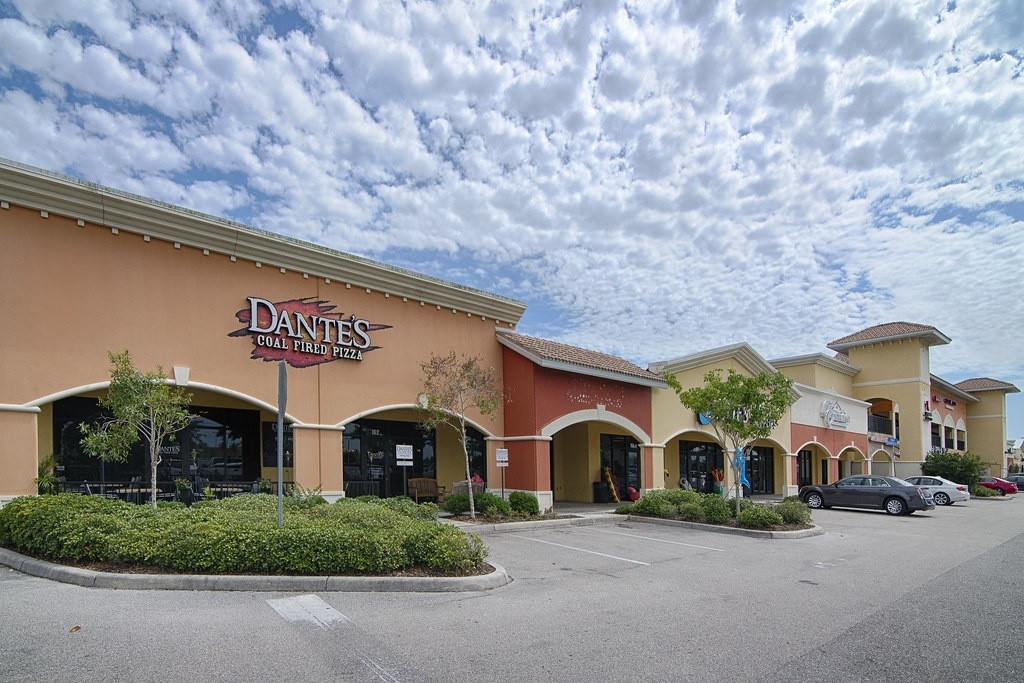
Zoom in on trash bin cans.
[592,481,610,504]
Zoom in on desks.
[202,488,243,499]
[117,489,162,505]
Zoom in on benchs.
[442,480,484,501]
[408,478,446,504]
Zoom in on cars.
[1001,475,1024,491]
[901,476,970,505]
[977,476,1018,497]
[798,474,936,516]
